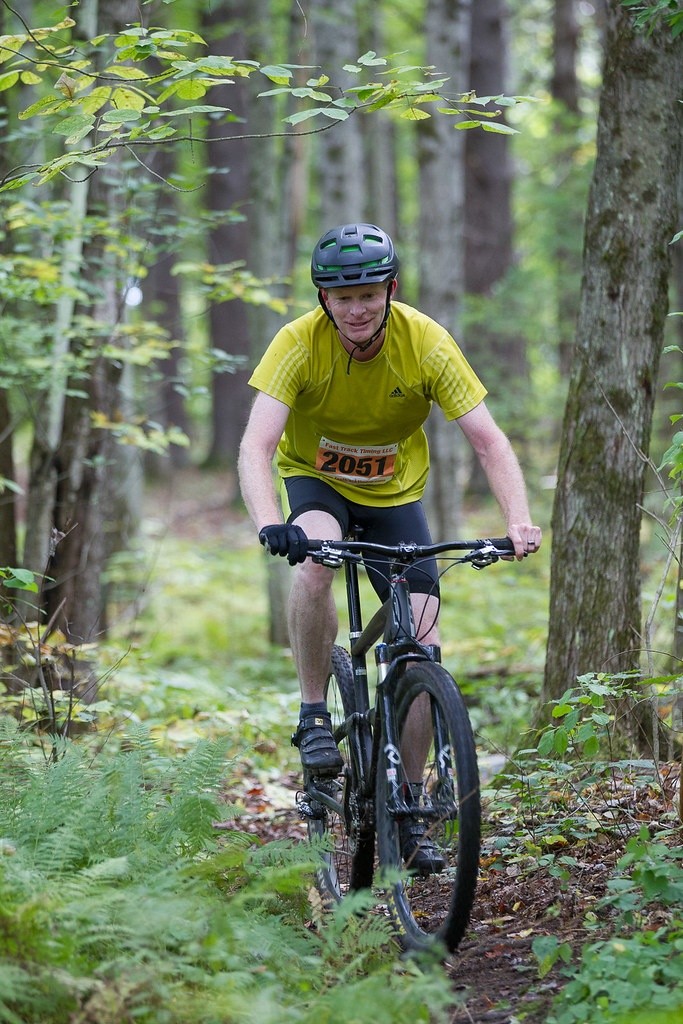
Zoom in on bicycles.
[260,524,539,961]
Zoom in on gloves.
[259,524,308,566]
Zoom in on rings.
[529,541,535,545]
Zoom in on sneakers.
[290,709,344,769]
[398,813,444,873]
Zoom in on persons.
[238,224,541,874]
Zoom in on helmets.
[311,223,401,289]
